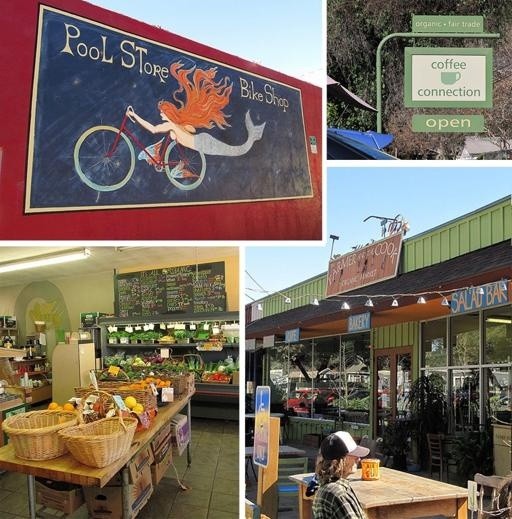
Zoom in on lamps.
[262,280,511,311]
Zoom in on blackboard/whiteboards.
[114,261,226,317]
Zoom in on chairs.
[427,433,457,482]
[273,457,308,519]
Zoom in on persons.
[311,430,368,519]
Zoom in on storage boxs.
[34,414,189,519]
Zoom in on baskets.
[73,367,196,419]
[57,389,138,467]
[1,409,78,459]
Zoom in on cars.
[278,370,413,422]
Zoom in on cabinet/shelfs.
[0,327,52,446]
[96,312,239,420]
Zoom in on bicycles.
[70,102,210,195]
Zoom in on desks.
[244,412,285,421]
[0,388,196,519]
[288,467,480,519]
[245,446,306,481]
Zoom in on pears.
[123,396,143,415]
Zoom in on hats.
[320,430,370,459]
